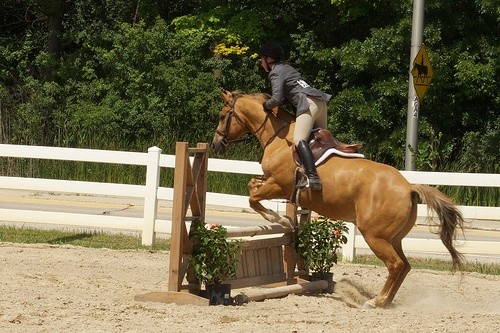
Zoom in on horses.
[211,88,472,308]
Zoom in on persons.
[258,42,332,190]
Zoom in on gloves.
[262,100,274,113]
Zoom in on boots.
[295,138,322,190]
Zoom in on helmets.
[258,42,285,60]
